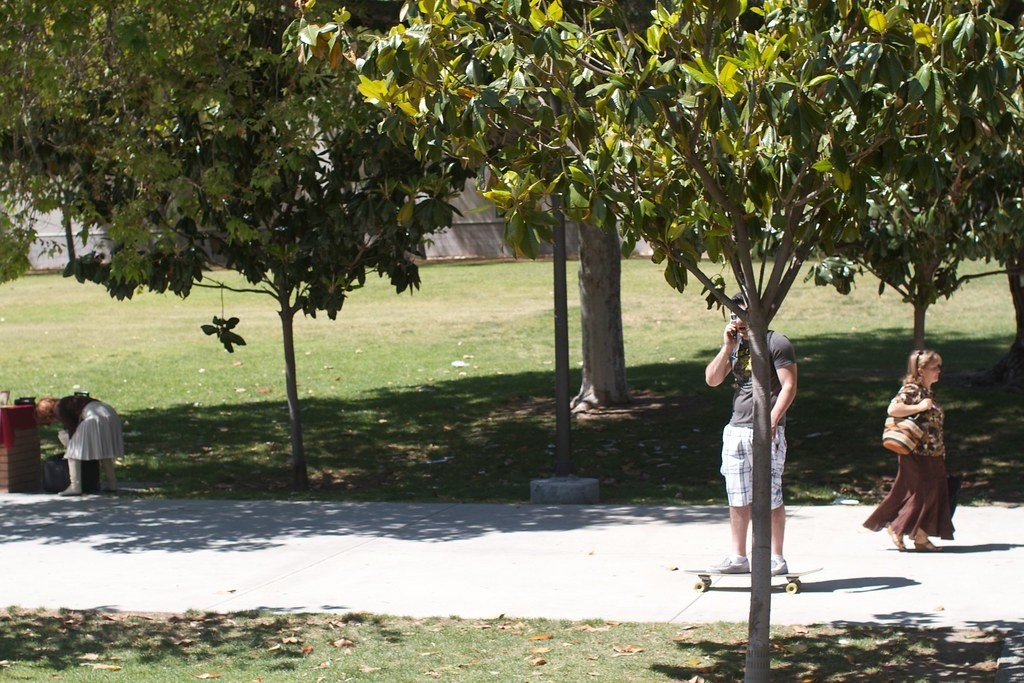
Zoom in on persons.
[34,395,123,496]
[705,291,798,574]
[886,350,950,553]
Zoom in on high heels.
[914,540,943,552]
[887,524,907,551]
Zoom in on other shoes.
[706,556,750,574]
[101,484,118,494]
[770,558,788,576]
[58,486,82,496]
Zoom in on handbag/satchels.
[882,386,924,455]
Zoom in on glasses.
[915,349,924,376]
[737,326,747,333]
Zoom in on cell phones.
[732,326,737,337]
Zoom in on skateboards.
[672,565,824,594]
